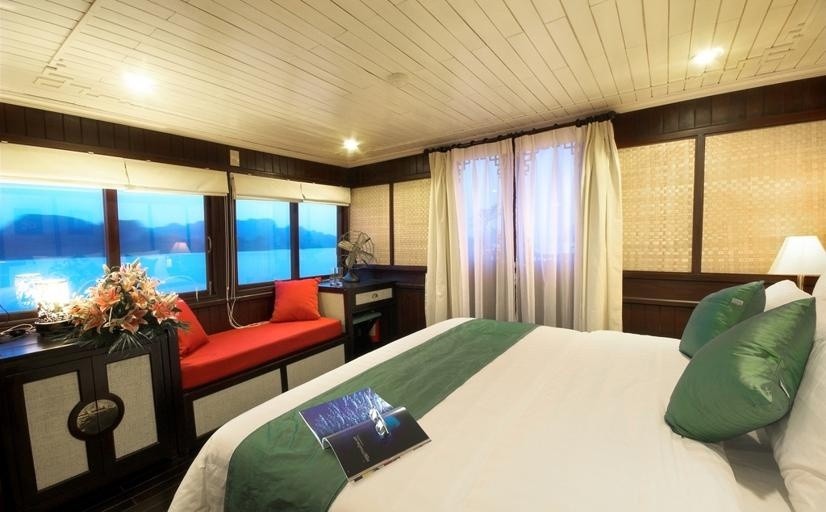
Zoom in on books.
[299,386,431,484]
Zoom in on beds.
[167,273,826,512]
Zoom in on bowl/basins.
[32,318,73,336]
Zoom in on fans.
[335,231,375,283]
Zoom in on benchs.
[178,316,352,468]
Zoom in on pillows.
[679,279,766,358]
[173,298,210,360]
[664,297,816,444]
[268,277,322,324]
[764,339,826,512]
[764,279,812,311]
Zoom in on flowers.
[63,259,192,356]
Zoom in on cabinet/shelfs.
[0,312,180,510]
[318,276,399,363]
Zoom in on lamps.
[767,236,826,291]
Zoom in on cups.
[333,267,345,285]
[328,276,336,286]
